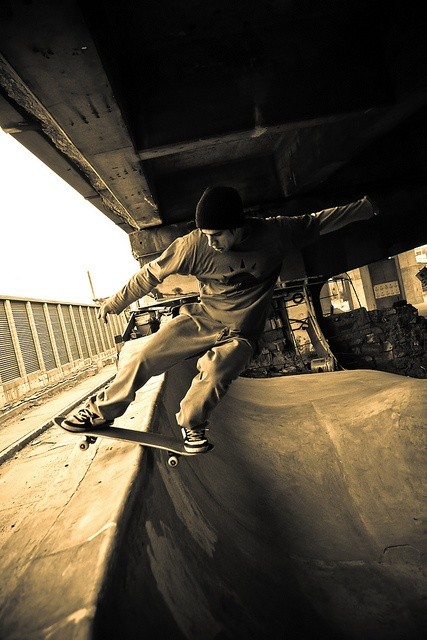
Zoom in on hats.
[194,183,247,231]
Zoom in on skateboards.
[52,417,216,468]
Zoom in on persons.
[60,182,380,453]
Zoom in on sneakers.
[61,403,116,433]
[181,422,210,453]
